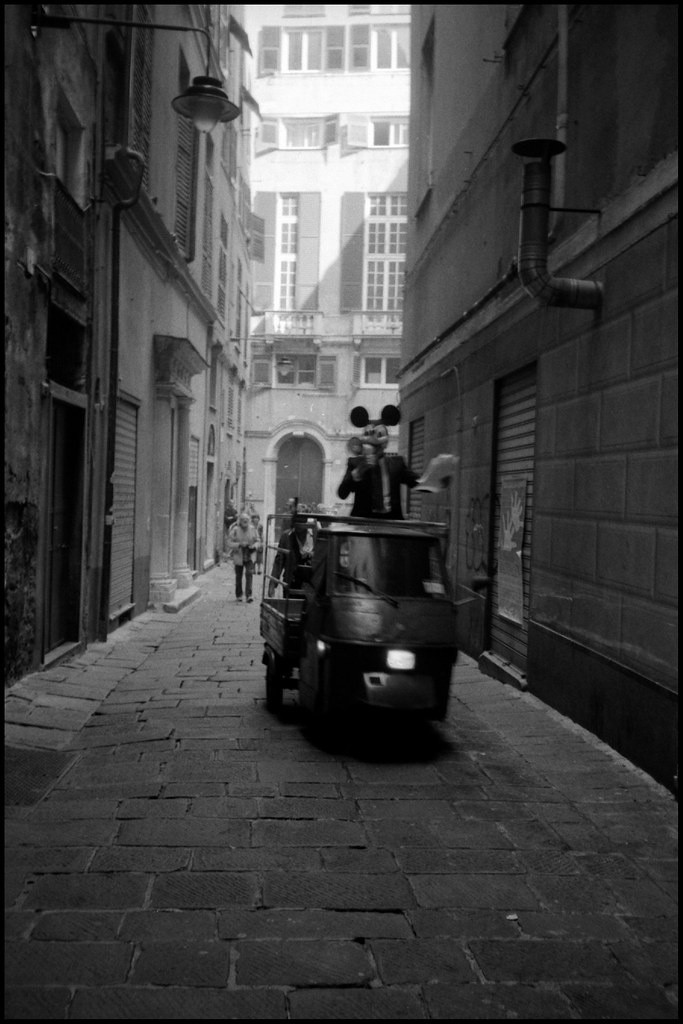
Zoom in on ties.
[379,458,391,512]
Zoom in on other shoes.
[237,597,242,601]
[246,596,253,603]
[257,571,261,575]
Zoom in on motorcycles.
[259,511,459,766]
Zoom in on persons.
[337,404,452,521]
[251,512,263,575]
[226,513,261,603]
[224,499,237,529]
[281,498,329,531]
[267,516,314,598]
